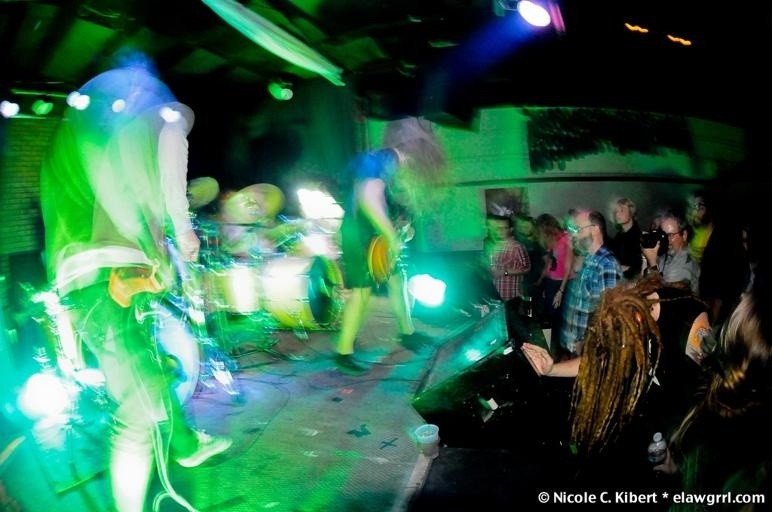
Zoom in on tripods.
[193,219,310,405]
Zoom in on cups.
[415,423,441,460]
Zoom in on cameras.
[641,229,669,257]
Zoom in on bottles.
[647,431,668,480]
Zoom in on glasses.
[668,229,682,240]
[578,224,595,233]
[695,202,706,210]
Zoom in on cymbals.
[226,183,284,231]
[186,175,219,209]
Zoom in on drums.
[211,260,260,318]
[258,251,343,337]
[228,232,276,264]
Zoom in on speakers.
[410,296,552,449]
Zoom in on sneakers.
[176,432,231,468]
[336,354,367,377]
[401,333,434,345]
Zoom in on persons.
[404,173,772,512]
[36,8,232,511]
[331,117,443,374]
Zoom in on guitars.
[367,212,413,287]
